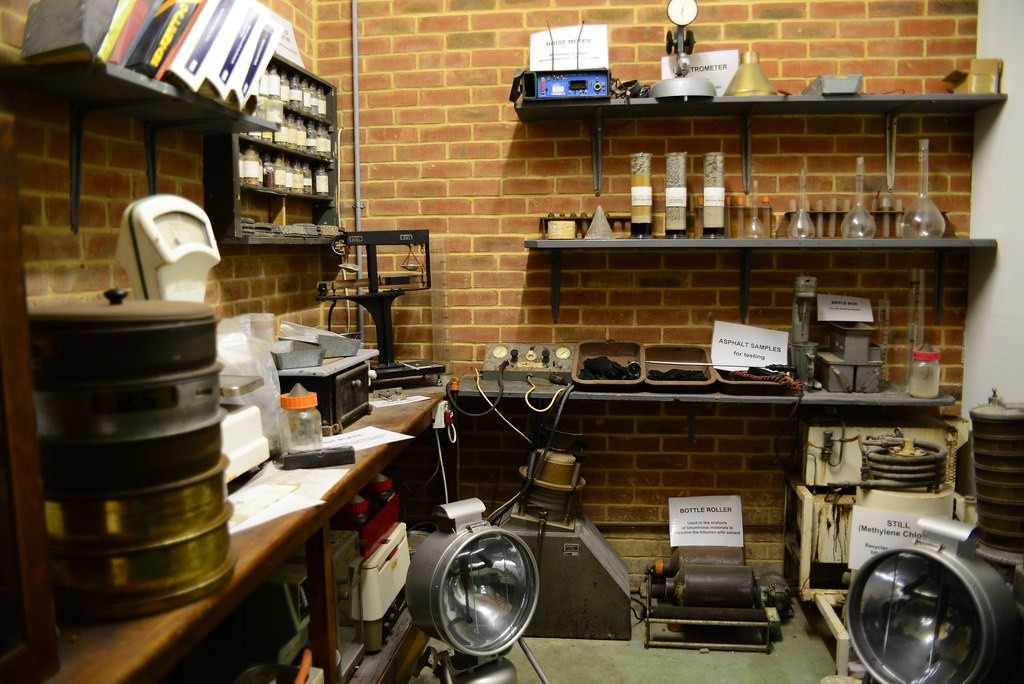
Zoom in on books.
[20,1,283,115]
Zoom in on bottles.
[840,157,876,238]
[240,63,332,197]
[786,168,815,238]
[744,179,766,237]
[909,343,941,398]
[277,383,323,456]
[901,139,946,239]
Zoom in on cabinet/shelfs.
[202,52,340,245]
[523,89,1008,322]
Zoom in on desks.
[53,374,453,684]
[456,371,956,584]
